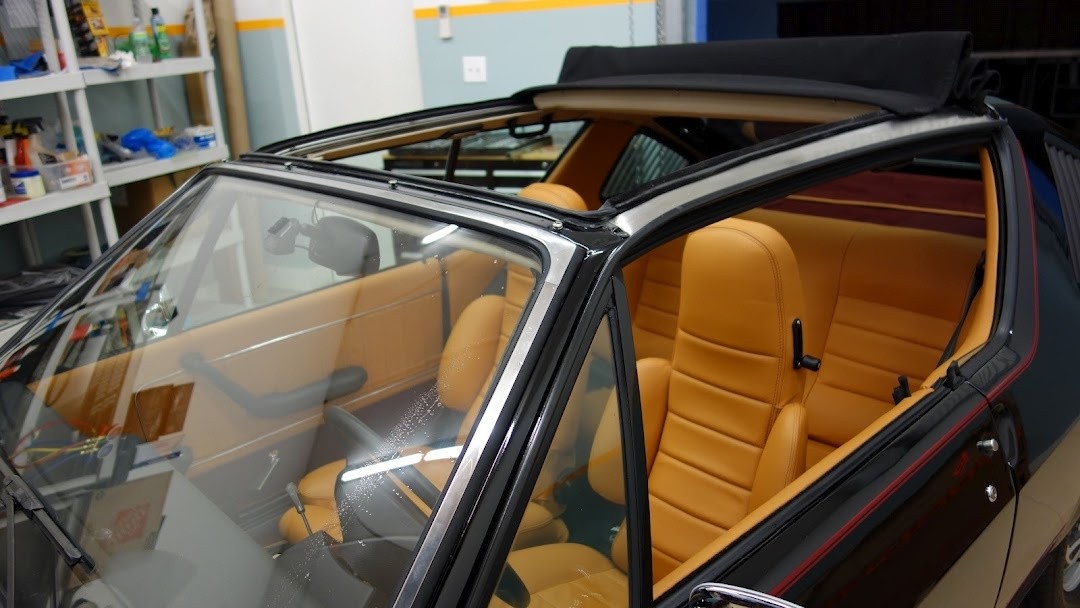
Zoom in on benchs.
[577,179,993,467]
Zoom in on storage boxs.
[128,164,199,221]
[67,0,109,59]
[36,150,95,191]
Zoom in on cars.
[0,30,1079,608]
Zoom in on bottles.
[9,170,48,199]
[129,17,153,65]
[150,8,172,60]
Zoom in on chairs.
[486,217,820,608]
[278,181,617,567]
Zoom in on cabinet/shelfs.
[0,0,252,357]
